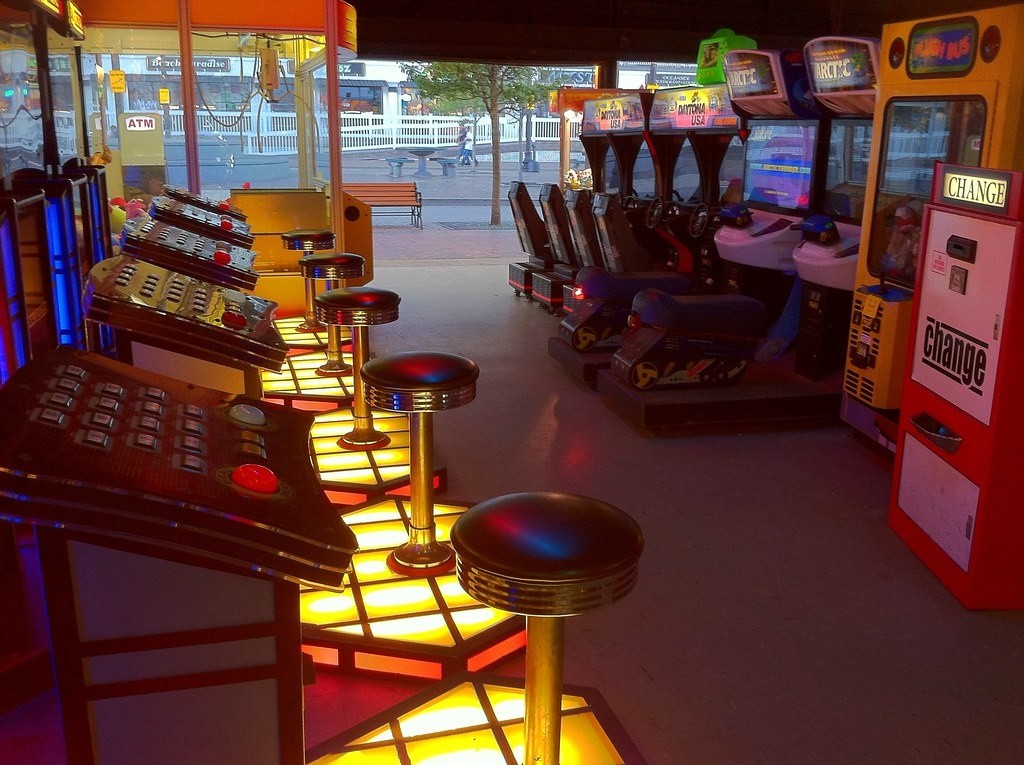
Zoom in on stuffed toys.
[875,206,920,281]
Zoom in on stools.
[298,253,366,378]
[448,491,648,764]
[311,286,403,452]
[385,158,416,178]
[360,350,481,579]
[429,158,458,177]
[281,228,338,335]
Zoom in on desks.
[405,147,439,178]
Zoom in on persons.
[457,126,478,166]
[455,123,467,162]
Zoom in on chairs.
[562,186,603,271]
[538,183,580,268]
[591,191,670,286]
[507,181,550,258]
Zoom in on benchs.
[341,181,424,229]
